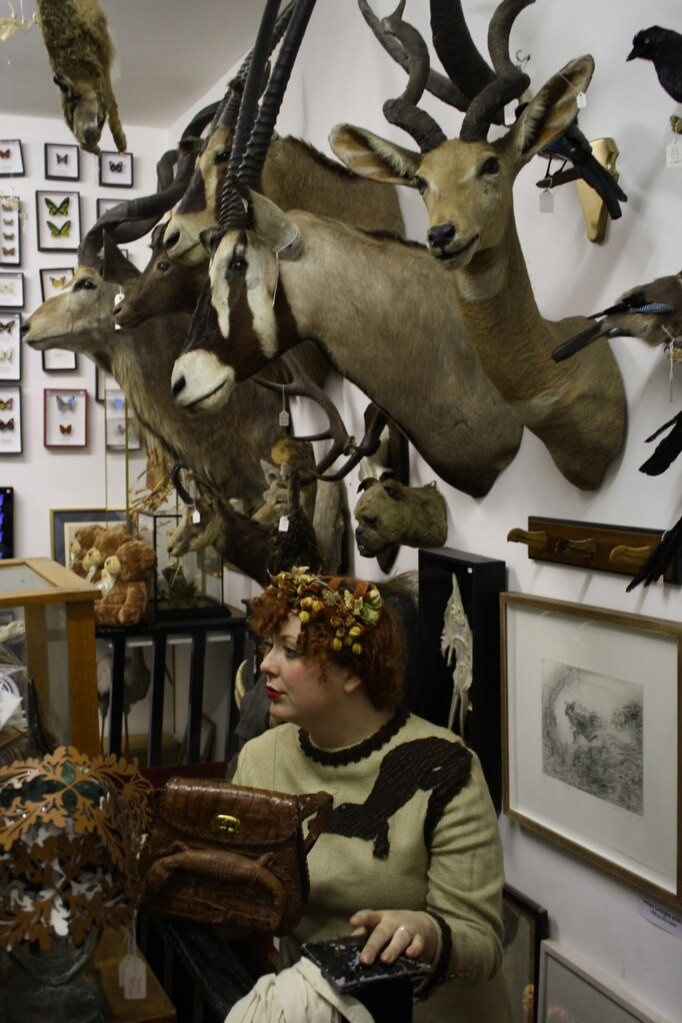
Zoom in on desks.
[94,598,245,767]
[0,555,103,770]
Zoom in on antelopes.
[20,0,682,589]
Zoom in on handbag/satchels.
[131,777,333,936]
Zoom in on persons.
[231,576,514,1022]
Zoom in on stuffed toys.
[71,523,155,627]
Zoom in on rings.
[402,926,412,936]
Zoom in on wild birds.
[624,24,682,105]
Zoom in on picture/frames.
[96,198,128,220]
[501,881,676,1023]
[0,385,23,454]
[44,142,80,181]
[0,196,21,265]
[0,139,24,175]
[98,150,133,186]
[104,388,141,451]
[0,248,130,401]
[497,591,682,908]
[35,190,81,250]
[43,388,87,448]
[49,508,139,567]
